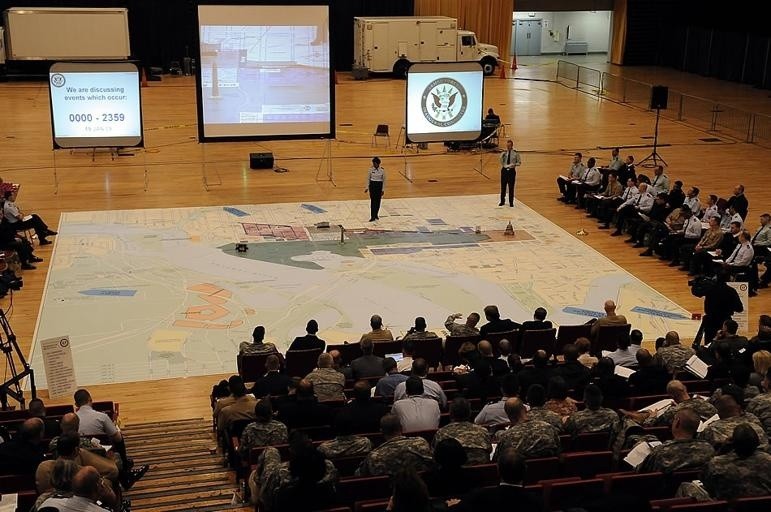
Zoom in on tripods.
[634,109,668,167]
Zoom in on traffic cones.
[138,68,149,86]
[508,54,519,70]
[499,64,506,79]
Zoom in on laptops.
[384,352,404,366]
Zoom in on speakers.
[250,153,274,170]
[650,84,668,109]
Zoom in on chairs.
[212,323,771,512]
[732,255,759,295]
[372,125,390,148]
[0,210,34,272]
[0,401,120,512]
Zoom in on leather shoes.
[40,231,57,246]
[21,256,42,269]
[556,198,701,276]
[122,464,149,490]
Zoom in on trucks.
[352,14,501,80]
[0,4,131,81]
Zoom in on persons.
[288,320,325,352]
[703,271,744,348]
[365,157,386,222]
[402,317,438,341]
[1,190,58,301]
[480,306,521,339]
[349,340,447,435]
[452,339,594,428]
[353,395,562,511]
[1,388,149,512]
[253,411,373,510]
[348,338,388,378]
[573,314,770,418]
[212,352,349,468]
[499,140,522,207]
[444,312,481,337]
[607,396,770,500]
[562,382,622,435]
[585,300,627,336]
[360,314,393,344]
[558,147,770,295]
[485,108,501,136]
[238,326,279,355]
[523,307,553,330]
